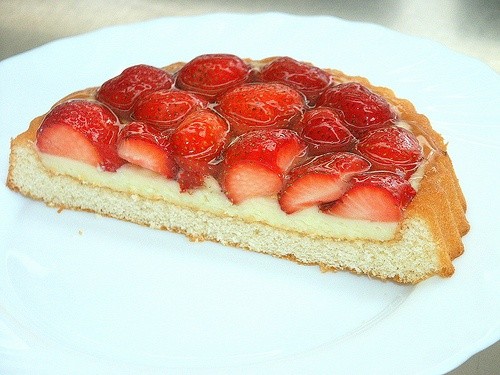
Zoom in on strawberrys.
[36,53,422,222]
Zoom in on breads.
[4,57,470,286]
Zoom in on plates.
[0,10,499,375]
